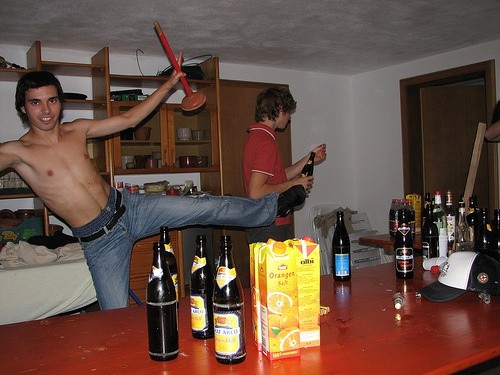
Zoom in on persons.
[243,86,326,245]
[0,49,305,310]
[484,120,500,142]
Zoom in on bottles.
[389,199,416,279]
[159,226,180,309]
[212,233,246,364]
[421,191,500,262]
[190,235,214,340]
[422,256,448,270]
[393,292,406,309]
[431,262,449,276]
[146,241,180,361]
[300,152,315,178]
[332,211,351,282]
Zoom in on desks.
[0,234,500,375]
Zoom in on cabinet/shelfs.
[0,39,226,306]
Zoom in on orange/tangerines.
[267,292,300,352]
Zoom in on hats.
[419,250,500,303]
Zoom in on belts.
[74,188,127,243]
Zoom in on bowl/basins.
[144,183,166,193]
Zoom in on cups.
[133,127,151,141]
[122,153,208,169]
[177,127,206,141]
[166,186,197,196]
[114,182,146,194]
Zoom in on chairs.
[310,204,388,274]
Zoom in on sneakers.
[276,183,306,217]
